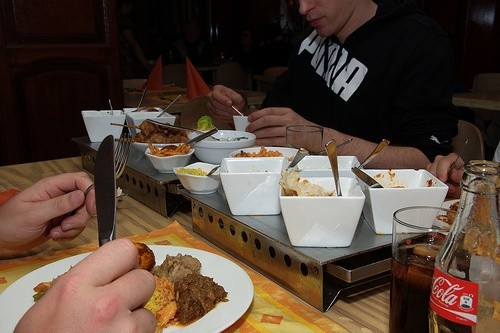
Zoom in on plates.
[0,244,254,332]
[432,199,460,229]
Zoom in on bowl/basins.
[293,155,365,170]
[279,170,366,247]
[81,111,126,143]
[123,106,176,139]
[228,147,299,163]
[173,161,221,195]
[232,116,250,131]
[187,130,256,164]
[134,142,182,155]
[356,168,450,234]
[144,146,195,173]
[220,158,288,215]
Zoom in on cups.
[388,206,457,333]
[286,125,324,156]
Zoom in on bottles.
[428,159,500,333]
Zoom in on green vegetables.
[196,115,216,130]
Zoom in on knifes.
[94,134,116,248]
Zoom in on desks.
[454,88,500,127]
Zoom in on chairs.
[458,118,483,163]
[474,72,500,120]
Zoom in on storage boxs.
[77,104,452,250]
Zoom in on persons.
[0,172,159,333]
[118,0,500,199]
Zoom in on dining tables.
[0,152,405,333]
